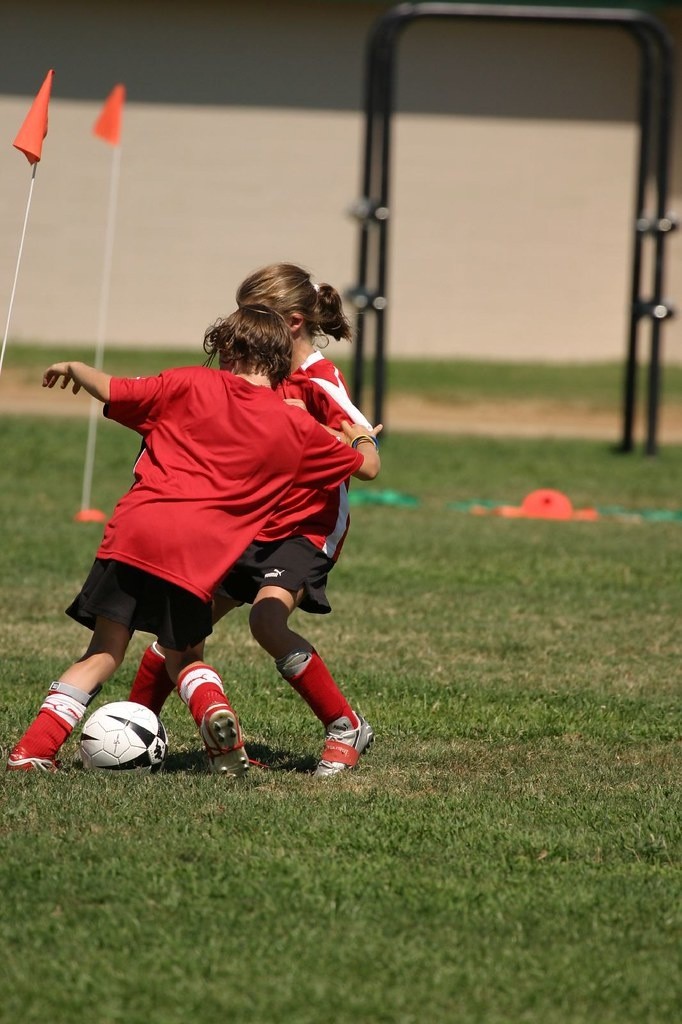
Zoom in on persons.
[7,304,382,771]
[129,264,374,774]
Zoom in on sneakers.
[6,744,69,776]
[200,700,251,779]
[314,710,374,776]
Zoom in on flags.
[93,83,126,144]
[13,70,53,165]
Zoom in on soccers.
[77,700,170,774]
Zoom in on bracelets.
[350,434,380,453]
